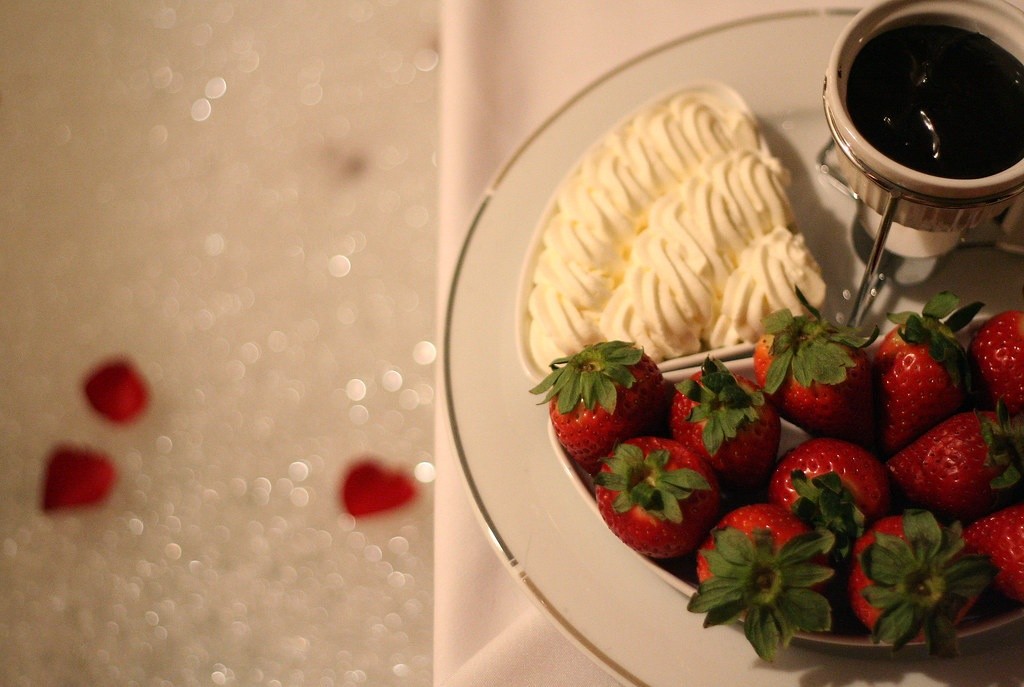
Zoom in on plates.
[441,11,1021,686]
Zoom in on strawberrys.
[528,293,1023,661]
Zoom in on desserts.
[519,82,826,384]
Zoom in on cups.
[822,1,1023,260]
